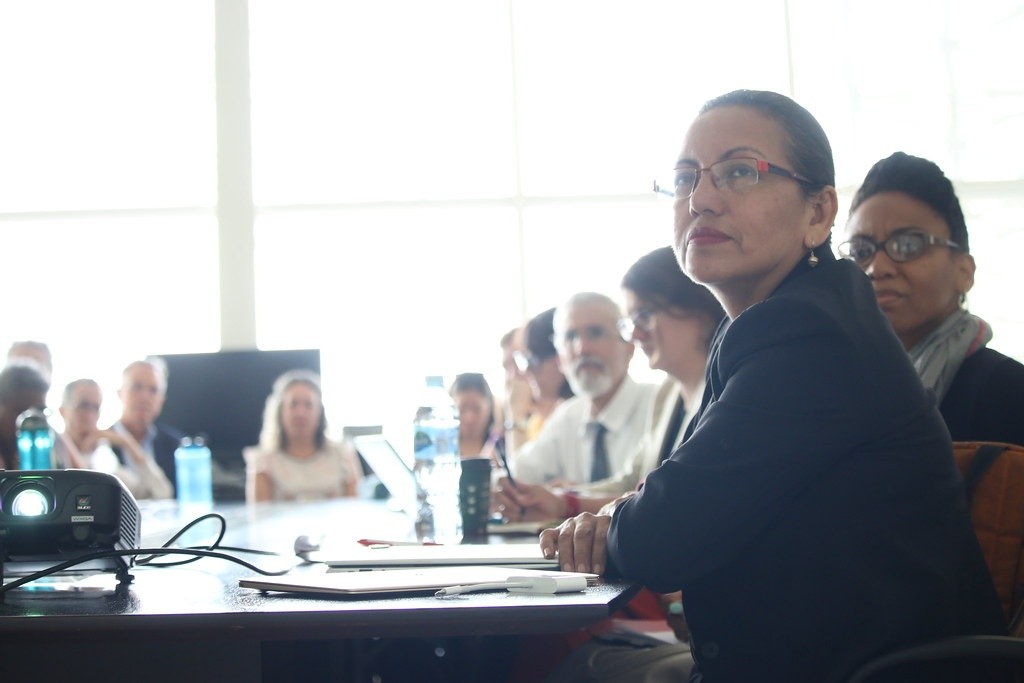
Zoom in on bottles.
[413,375,464,546]
[174,436,214,509]
[13,403,51,470]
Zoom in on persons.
[0,342,365,506]
[451,91,1024,683]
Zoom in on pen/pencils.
[358,538,443,546]
[495,439,525,517]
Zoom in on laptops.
[353,434,544,533]
[326,543,563,573]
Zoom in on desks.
[0,500,644,683]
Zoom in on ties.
[590,422,610,483]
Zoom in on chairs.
[849,634,1024,683]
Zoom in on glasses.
[619,299,686,342]
[63,401,99,414]
[652,155,817,200]
[836,229,961,269]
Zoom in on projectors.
[0,467,142,582]
